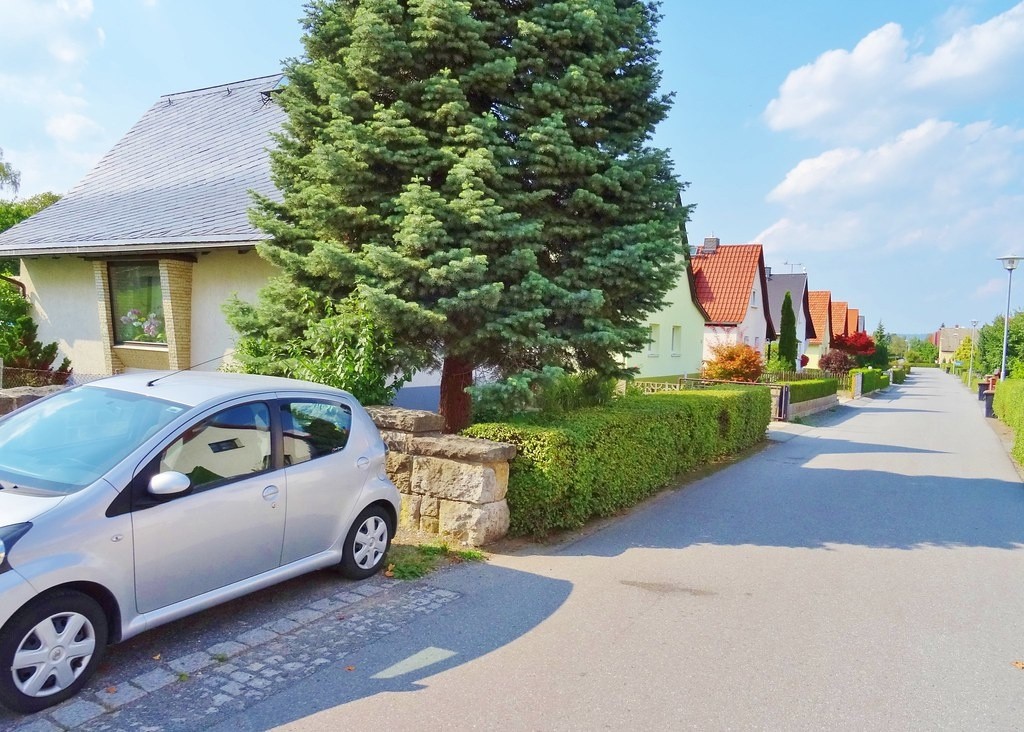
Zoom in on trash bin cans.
[978,382,990,401]
[983,389,996,418]
[946,367,951,373]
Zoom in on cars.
[0,368,402,710]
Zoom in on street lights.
[996,254,1024,382]
[968,319,980,392]
[953,333,959,374]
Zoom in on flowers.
[120,309,162,337]
